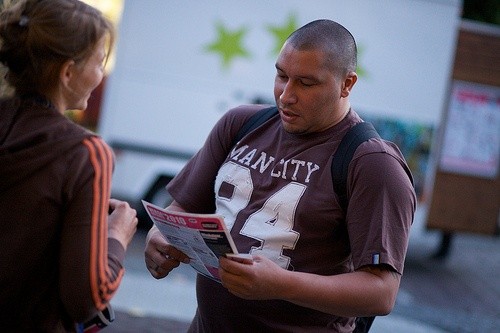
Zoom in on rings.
[149,264,160,273]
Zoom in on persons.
[139,18,416,332]
[0,0,136,333]
